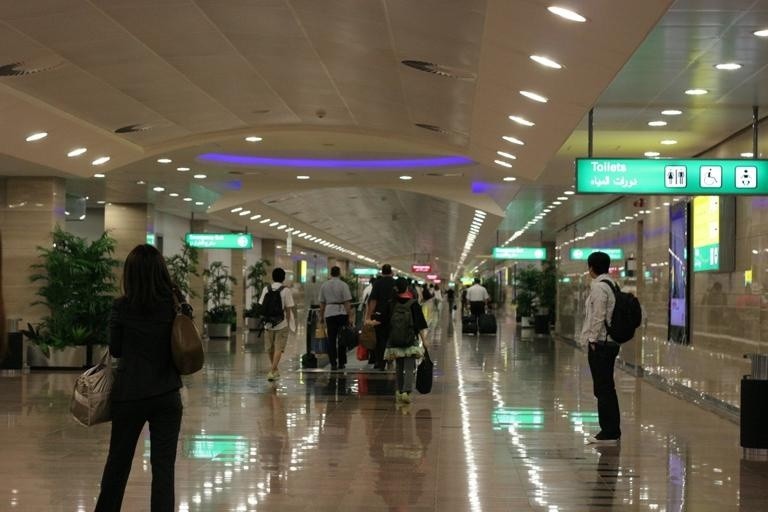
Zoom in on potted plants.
[203,259,236,339]
[243,256,274,329]
[17,223,122,371]
[516,265,568,335]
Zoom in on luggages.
[463,309,477,333]
[479,305,496,334]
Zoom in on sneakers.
[396,390,411,403]
[268,370,279,381]
[588,431,620,444]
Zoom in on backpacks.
[600,279,641,343]
[261,285,285,316]
[390,298,417,345]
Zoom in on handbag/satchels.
[372,312,390,332]
[416,360,432,394]
[171,313,204,374]
[71,361,118,428]
[315,328,328,339]
[361,327,376,350]
[357,346,369,360]
[344,327,358,350]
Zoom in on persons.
[578,250,624,447]
[445,286,454,312]
[255,266,295,382]
[358,264,434,404]
[94,243,183,512]
[460,277,492,317]
[434,284,442,311]
[318,266,354,370]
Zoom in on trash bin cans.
[0,317,24,378]
[534,306,550,338]
[740,352,768,462]
